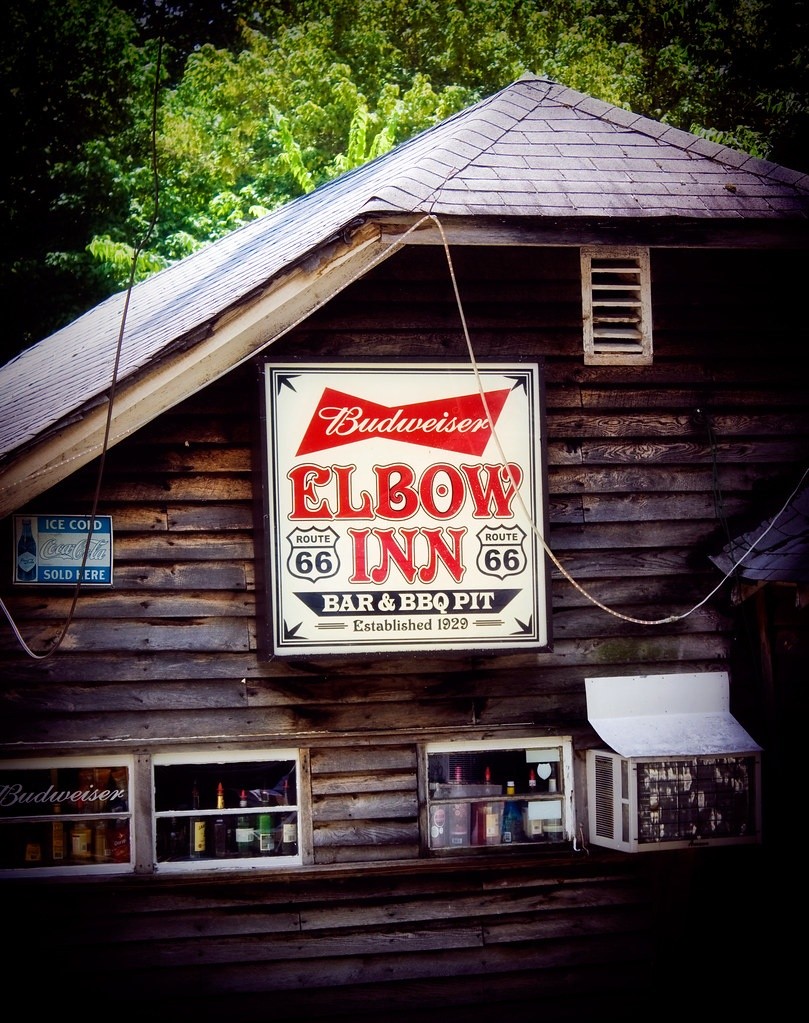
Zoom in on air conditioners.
[586,747,762,853]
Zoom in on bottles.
[474,768,502,845]
[523,769,543,842]
[502,781,523,844]
[277,781,297,855]
[213,783,229,857]
[258,792,274,854]
[545,763,563,840]
[24,801,129,861]
[234,790,254,853]
[449,767,470,847]
[75,768,128,811]
[430,782,447,846]
[189,789,206,860]
